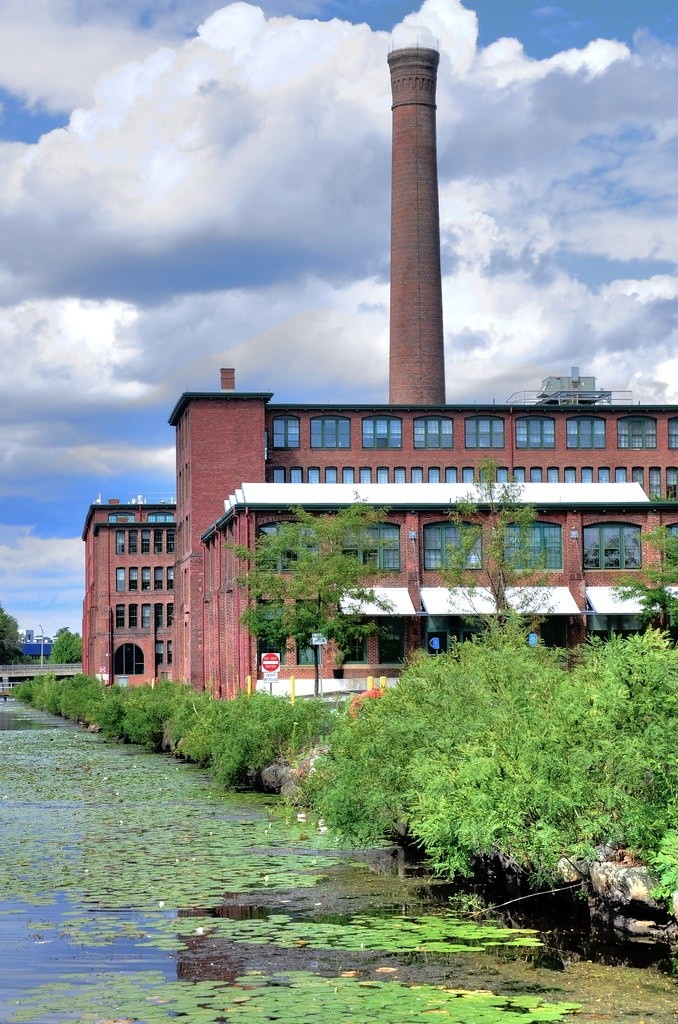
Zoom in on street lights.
[38,624,44,671]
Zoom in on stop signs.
[261,652,281,673]
